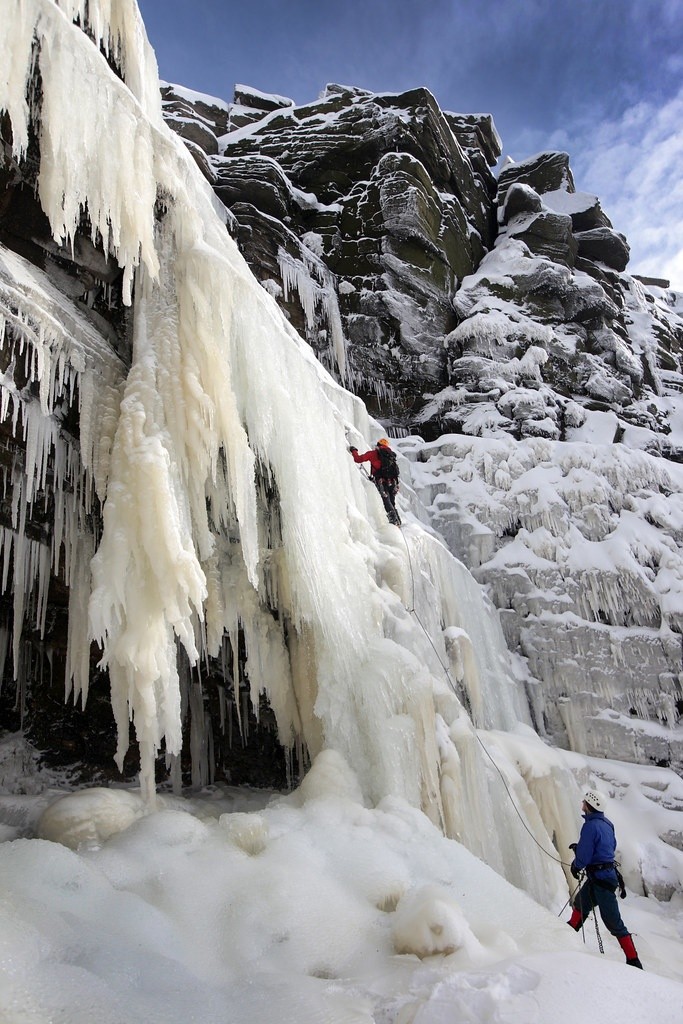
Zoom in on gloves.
[349,446,358,452]
[570,859,580,880]
[568,843,579,857]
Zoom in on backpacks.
[376,449,400,485]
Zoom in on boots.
[386,509,401,526]
[566,904,588,933]
[616,932,643,970]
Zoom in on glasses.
[376,442,382,446]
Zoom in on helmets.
[378,438,389,446]
[583,790,607,813]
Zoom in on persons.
[567,790,647,970]
[345,438,409,528]
